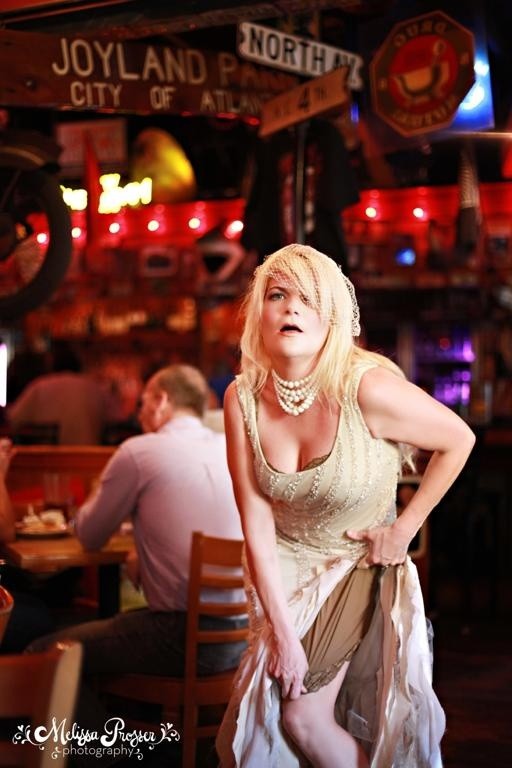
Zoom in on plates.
[17,523,71,539]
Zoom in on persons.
[76,364,246,757]
[211,243,476,768]
[8,344,105,449]
[0,430,18,543]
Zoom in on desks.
[3,533,138,620]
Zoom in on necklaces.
[270,367,323,415]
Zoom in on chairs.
[0,640,87,767]
[100,529,256,767]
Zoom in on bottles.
[412,331,478,426]
